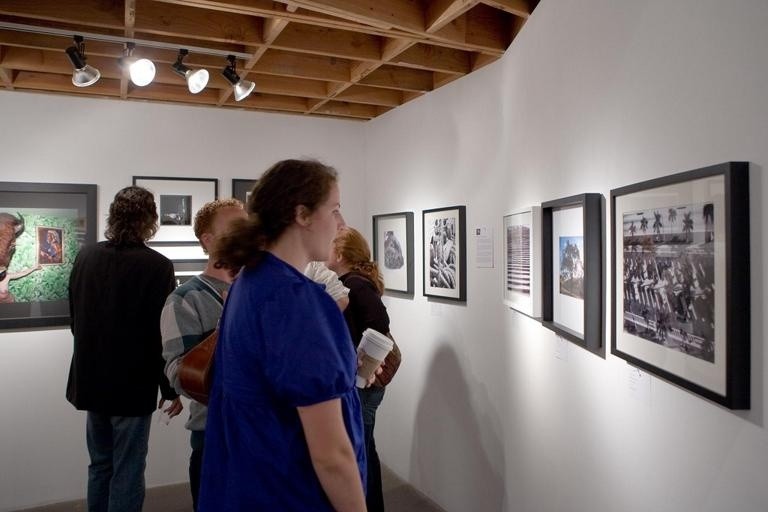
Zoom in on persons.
[41,228,63,264]
[197,157,365,511]
[304,257,350,313]
[64,182,184,511]
[322,224,401,510]
[160,197,247,511]
[384,230,401,259]
[429,219,456,288]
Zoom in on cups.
[352,328,394,389]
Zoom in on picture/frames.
[500,206,543,321]
[231,178,262,207]
[370,211,414,299]
[539,191,603,353]
[33,224,65,268]
[422,206,468,305]
[610,160,752,410]
[132,176,220,247]
[172,259,217,288]
[0,180,101,328]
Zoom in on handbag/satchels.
[177,326,222,407]
[371,329,402,390]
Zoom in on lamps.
[65,31,256,103]
